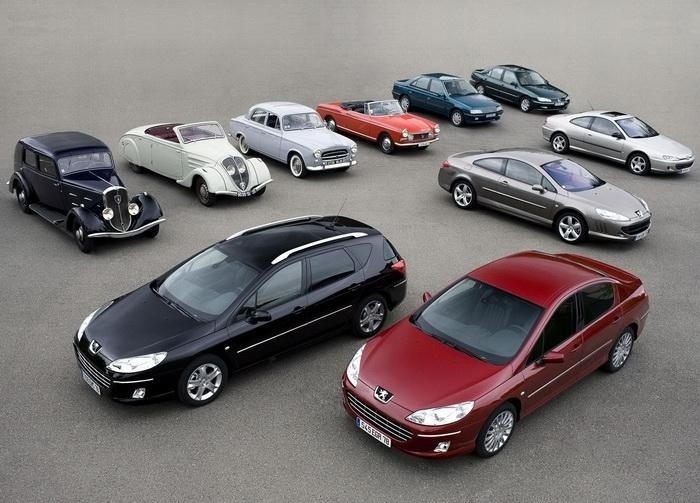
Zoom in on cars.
[541,109,695,175]
[437,146,652,243]
[391,73,502,126]
[341,251,651,461]
[74,214,406,408]
[8,128,167,253]
[228,101,359,178]
[469,64,568,111]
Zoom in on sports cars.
[118,113,274,206]
[316,98,441,154]
